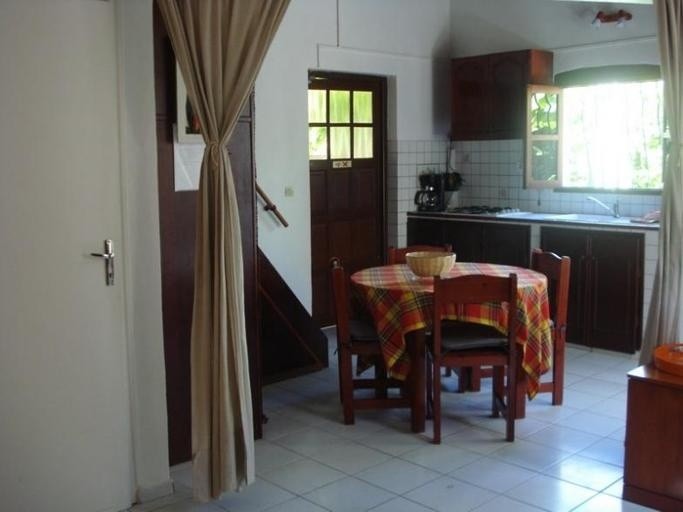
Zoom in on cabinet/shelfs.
[451,49,554,141]
[153,0,262,466]
[407,219,530,268]
[541,227,645,354]
[622,363,683,512]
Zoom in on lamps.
[590,10,632,29]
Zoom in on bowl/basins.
[405,250,457,280]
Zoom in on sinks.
[545,213,632,224]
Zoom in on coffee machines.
[415,173,444,211]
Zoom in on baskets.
[406,251,456,276]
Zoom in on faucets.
[587,196,621,218]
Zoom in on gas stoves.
[448,205,521,214]
